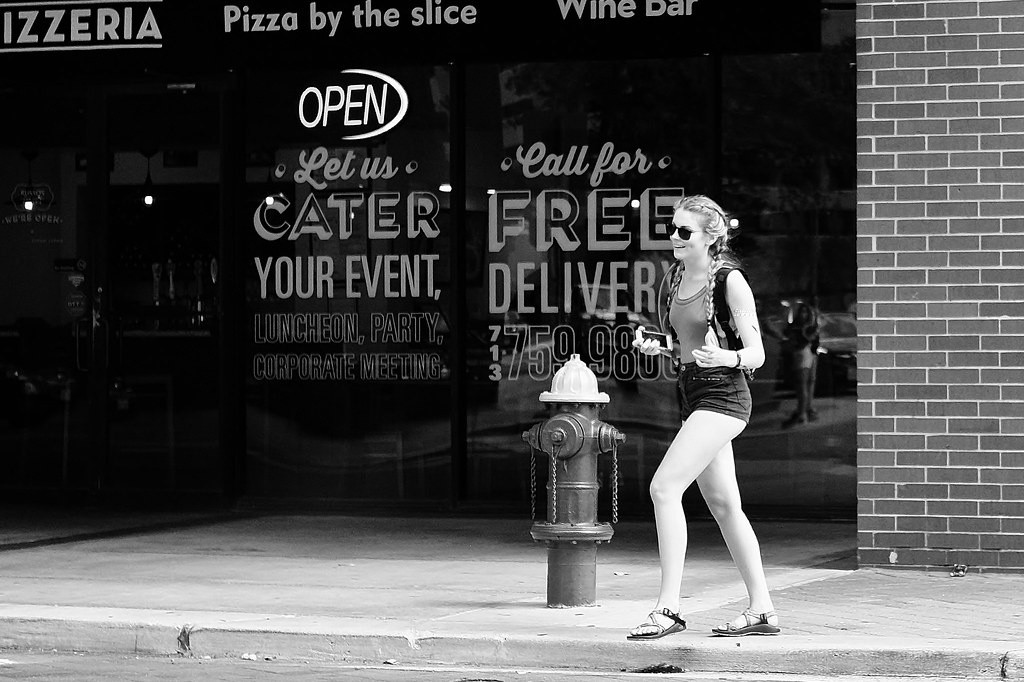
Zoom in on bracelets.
[731,350,741,368]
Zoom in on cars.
[810,313,857,396]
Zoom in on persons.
[626,194,780,641]
[781,283,819,429]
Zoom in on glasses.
[666,223,705,240]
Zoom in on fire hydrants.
[522,354,627,610]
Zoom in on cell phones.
[635,330,674,352]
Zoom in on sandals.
[626,608,686,640]
[711,609,780,637]
